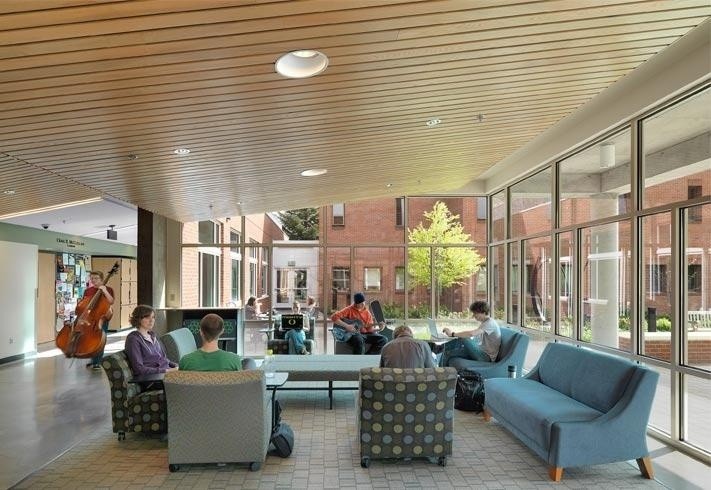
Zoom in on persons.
[245,297,263,320]
[178,313,256,372]
[125,305,178,390]
[306,295,315,313]
[279,301,311,355]
[331,292,388,355]
[428,301,502,367]
[85,271,115,370]
[379,325,437,369]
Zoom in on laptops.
[282,314,303,330]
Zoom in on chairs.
[359,365,457,469]
[333,323,371,355]
[267,317,315,355]
[446,328,530,379]
[100,327,273,473]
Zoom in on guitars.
[332,318,394,344]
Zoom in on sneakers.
[86,363,101,369]
[427,341,443,354]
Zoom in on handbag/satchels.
[270,423,294,457]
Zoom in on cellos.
[56,262,119,359]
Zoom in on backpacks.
[454,369,485,412]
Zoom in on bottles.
[265,349,275,379]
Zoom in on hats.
[354,292,365,304]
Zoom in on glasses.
[92,277,96,279]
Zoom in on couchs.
[482,342,661,482]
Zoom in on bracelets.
[452,331,455,337]
